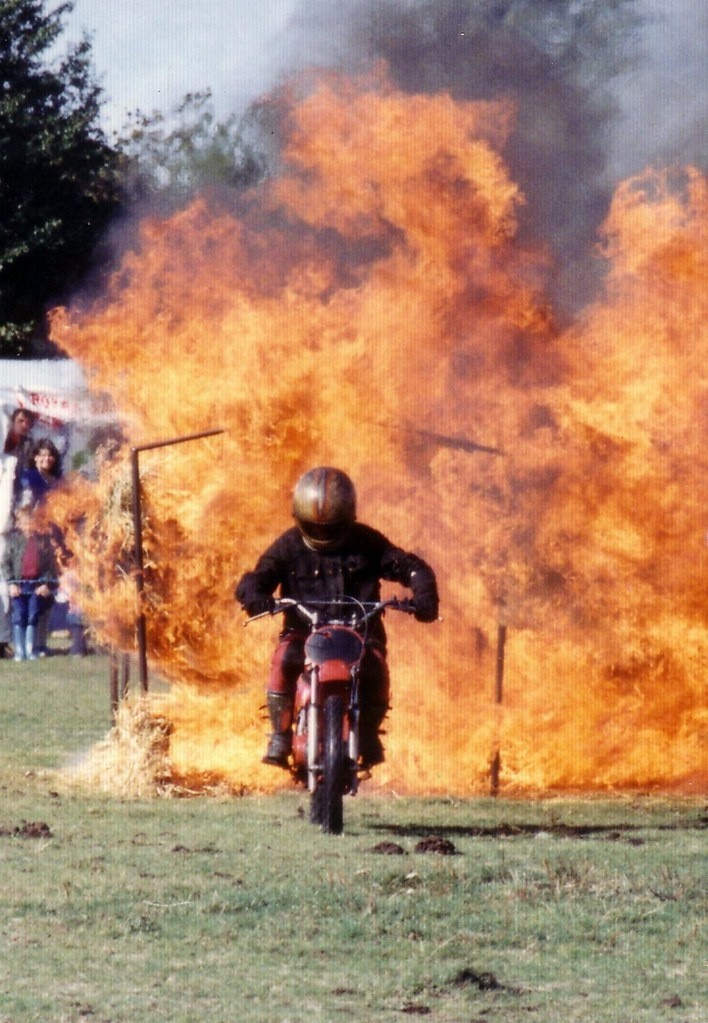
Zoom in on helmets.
[291,466,356,552]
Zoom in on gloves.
[243,587,275,617]
[408,589,439,623]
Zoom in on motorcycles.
[244,590,444,838]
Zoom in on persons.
[0,506,60,661]
[0,409,103,659]
[235,467,439,767]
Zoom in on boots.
[260,689,295,760]
[25,624,37,660]
[12,624,25,661]
[360,699,392,763]
